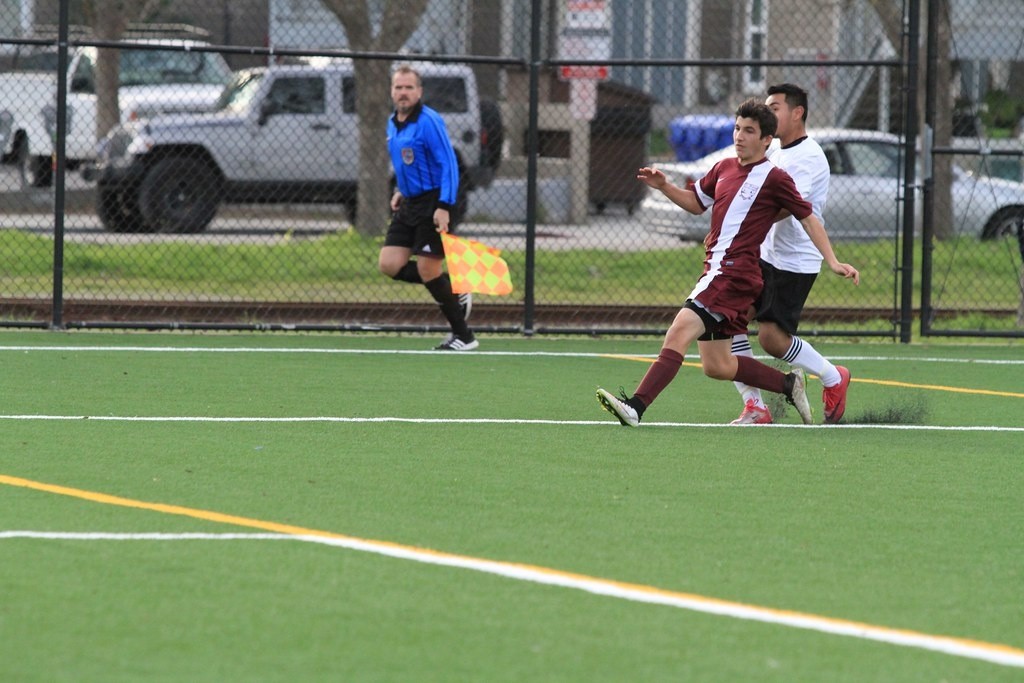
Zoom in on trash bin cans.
[669,114,737,164]
[589,79,658,215]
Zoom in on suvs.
[78,50,504,234]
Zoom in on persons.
[730,83,852,426]
[598,97,859,428]
[382,65,481,352]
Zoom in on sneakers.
[432,330,479,351]
[823,366,850,423]
[458,292,471,322]
[730,398,772,424]
[784,367,814,425]
[596,385,640,428]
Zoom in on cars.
[0,31,234,186]
[637,125,1024,252]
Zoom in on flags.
[442,227,513,298]
